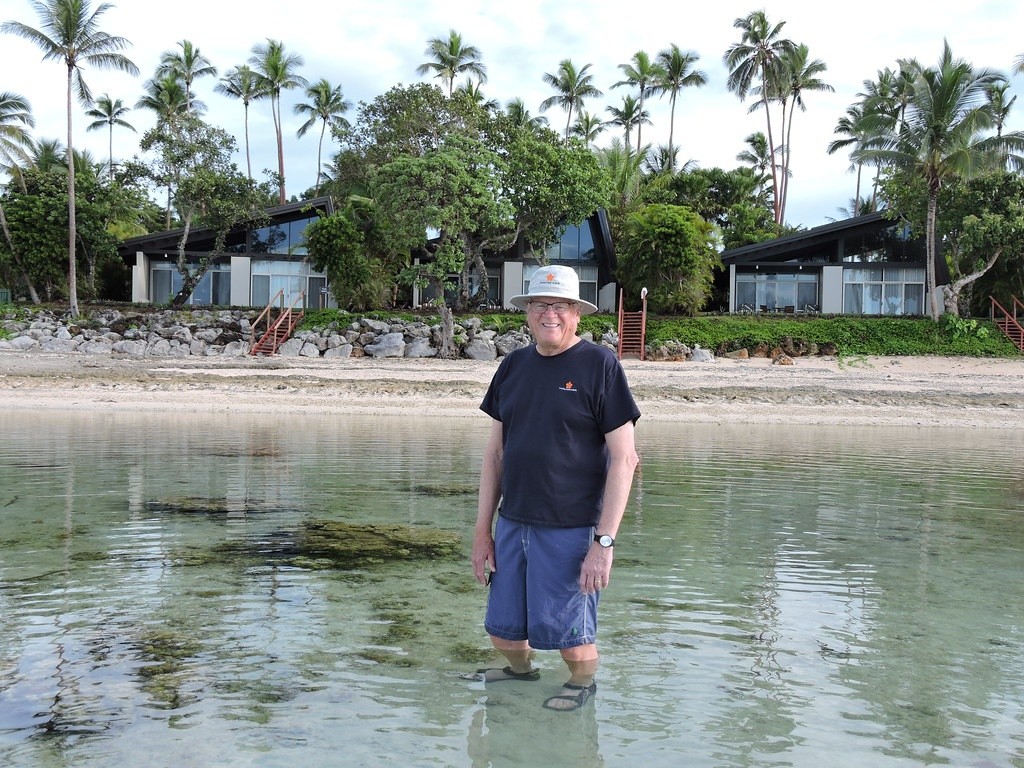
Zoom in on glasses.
[527,298,577,313]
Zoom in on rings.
[594,580,601,583]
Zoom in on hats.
[510,265,598,314]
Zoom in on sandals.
[459,665,540,684]
[542,678,597,713]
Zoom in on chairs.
[782,305,794,314]
[760,305,772,312]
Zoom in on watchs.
[593,531,615,549]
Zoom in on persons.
[460,264,641,713]
